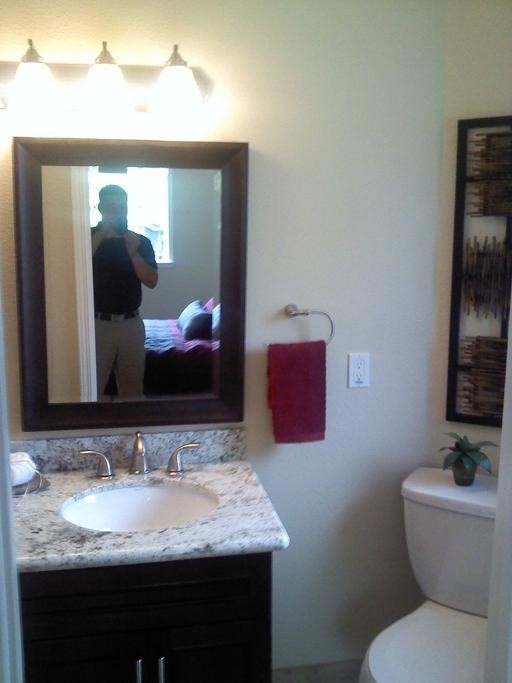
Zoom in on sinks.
[60,479,218,533]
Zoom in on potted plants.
[437,430,501,486]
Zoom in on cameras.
[114,216,127,235]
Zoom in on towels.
[211,303,222,341]
[267,341,326,444]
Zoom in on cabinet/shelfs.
[18,553,272,683]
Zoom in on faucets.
[129,431,150,474]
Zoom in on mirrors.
[11,133,249,433]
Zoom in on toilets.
[357,466,512,683]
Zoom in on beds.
[104,318,221,396]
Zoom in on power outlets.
[347,351,371,387]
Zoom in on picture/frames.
[445,115,512,429]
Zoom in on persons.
[91,185,157,400]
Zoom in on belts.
[94,311,140,321]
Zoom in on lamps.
[2,38,205,122]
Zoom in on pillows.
[178,300,212,339]
[204,296,218,312]
